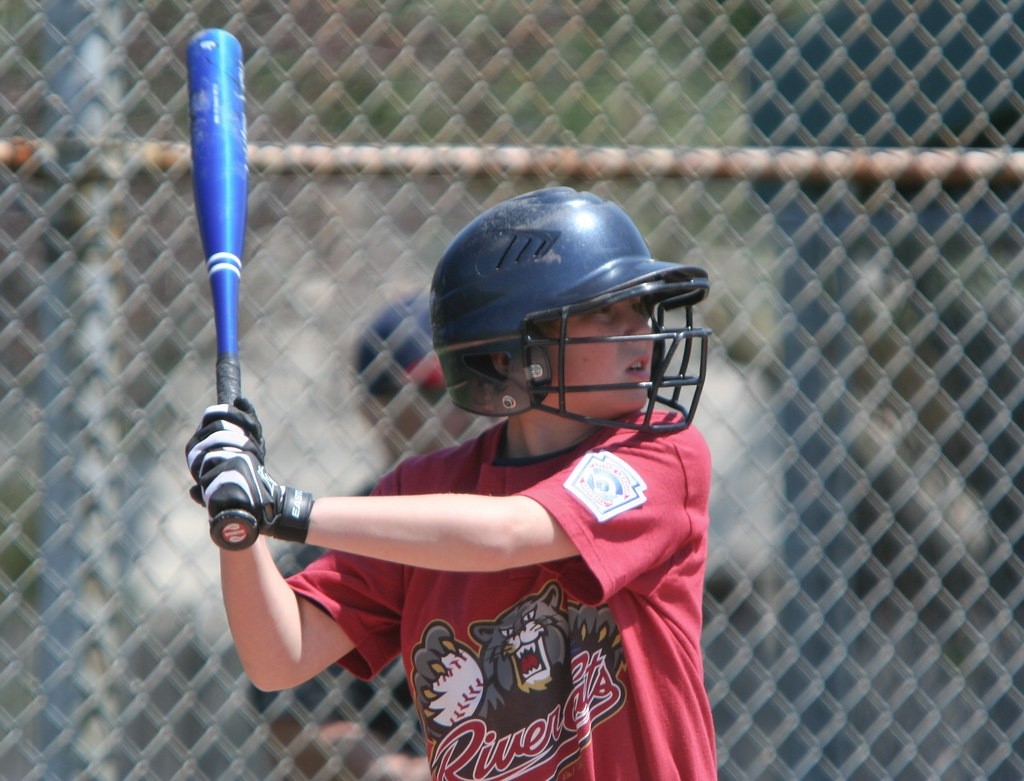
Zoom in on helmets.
[430,186,711,430]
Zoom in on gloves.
[191,448,313,544]
[184,398,267,483]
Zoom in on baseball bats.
[185,27,262,552]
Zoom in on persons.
[245,286,509,781]
[184,184,718,781]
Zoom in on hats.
[357,291,446,390]
